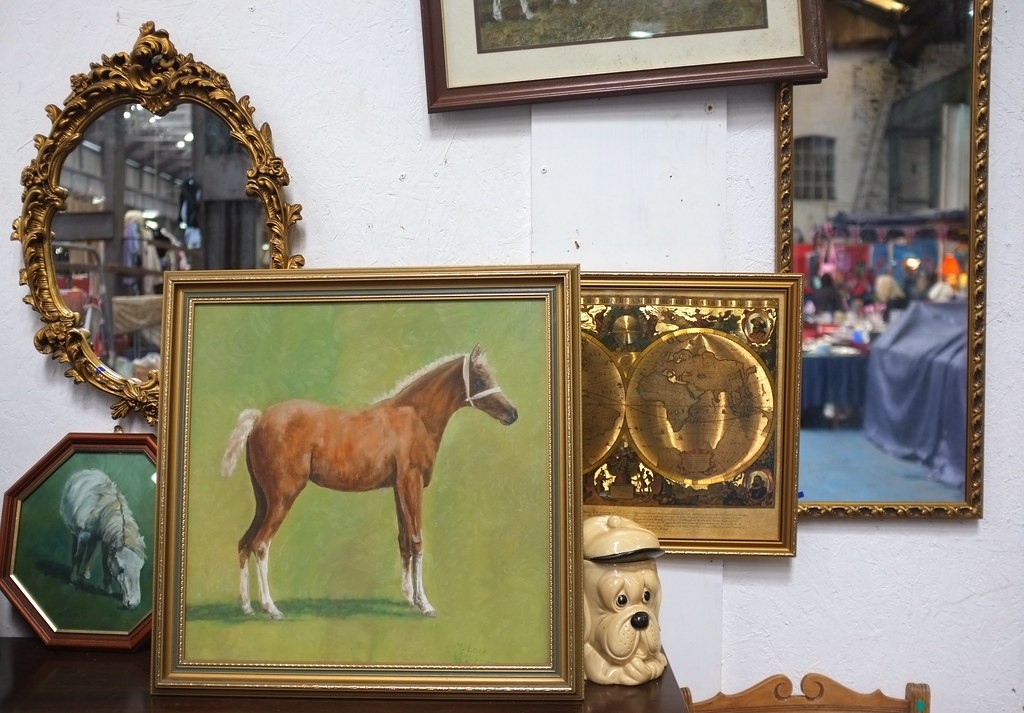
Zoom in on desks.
[804,352,869,428]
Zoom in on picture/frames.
[420,0,828,114]
[0,430,158,652]
[580,270,804,560]
[144,260,586,701]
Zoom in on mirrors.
[13,21,308,422]
[772,0,993,524]
